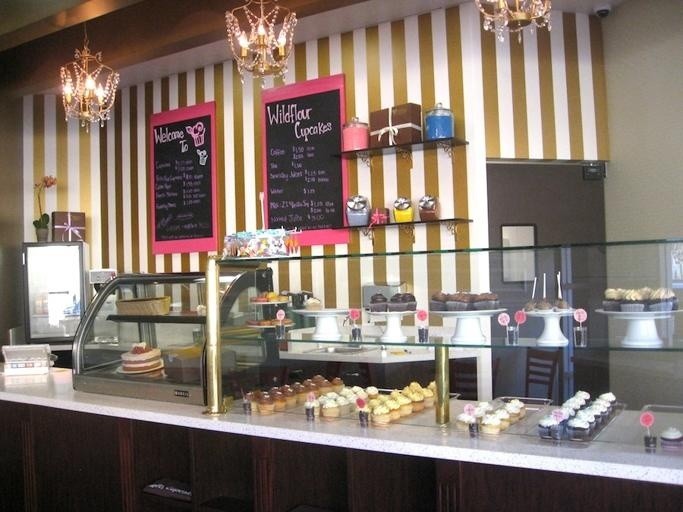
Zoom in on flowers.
[33,176,57,227]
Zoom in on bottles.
[418,194,441,221]
[346,195,370,226]
[424,101,455,141]
[33,293,49,314]
[342,117,369,153]
[393,197,414,223]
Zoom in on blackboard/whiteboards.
[148,101,219,255]
[261,73,350,246]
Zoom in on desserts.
[245,288,683,448]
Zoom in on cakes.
[122,343,162,371]
[117,295,169,316]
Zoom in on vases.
[36,227,48,242]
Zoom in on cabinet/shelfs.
[0,239,683,512]
[330,137,473,230]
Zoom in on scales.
[2,344,50,375]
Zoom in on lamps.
[58,24,120,132]
[475,0,552,43]
[224,0,298,87]
